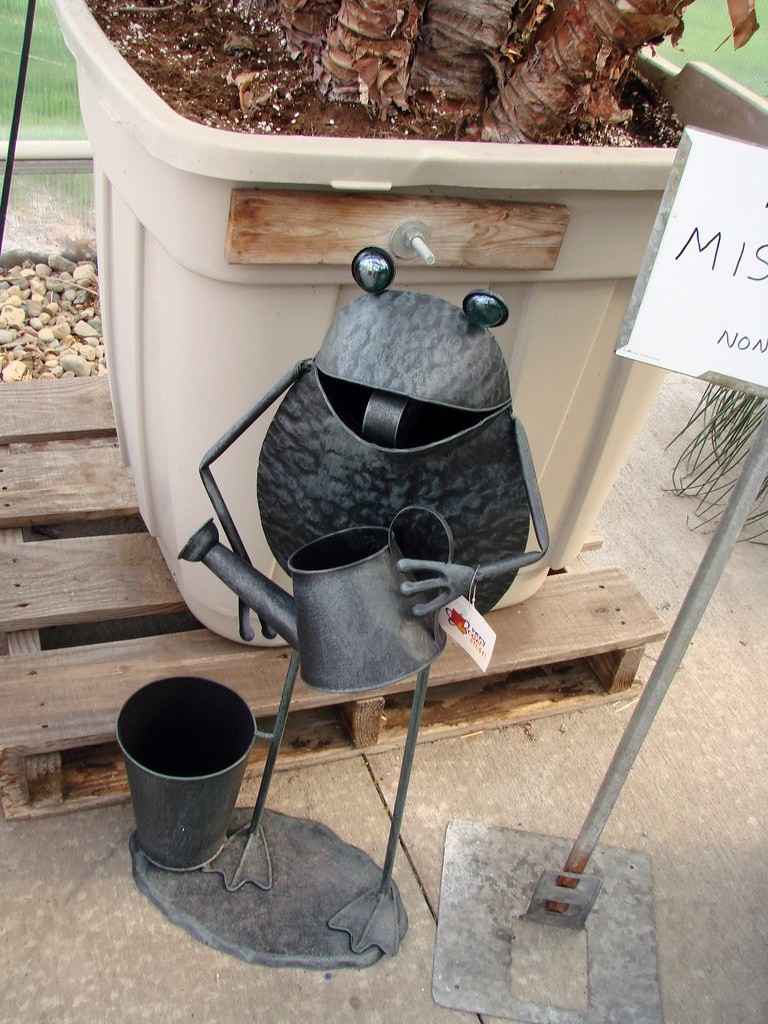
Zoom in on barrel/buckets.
[115,676,255,873]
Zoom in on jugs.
[176,503,454,692]
